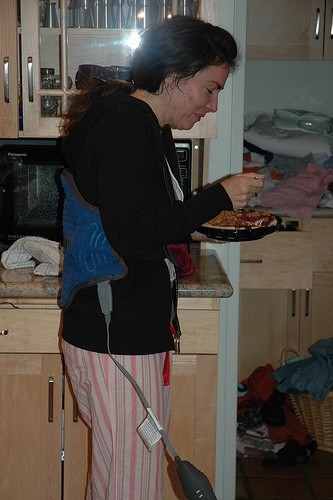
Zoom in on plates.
[195,211,284,243]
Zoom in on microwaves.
[0,138,210,255]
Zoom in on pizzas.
[201,210,277,229]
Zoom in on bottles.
[40,67,57,117]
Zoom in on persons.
[59,15,264,500]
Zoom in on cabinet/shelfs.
[0,0,218,139]
[0,296,221,500]
[246,0,333,61]
[237,216,333,382]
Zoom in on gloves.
[24,241,64,276]
[1,237,61,270]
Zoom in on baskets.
[282,348,333,453]
